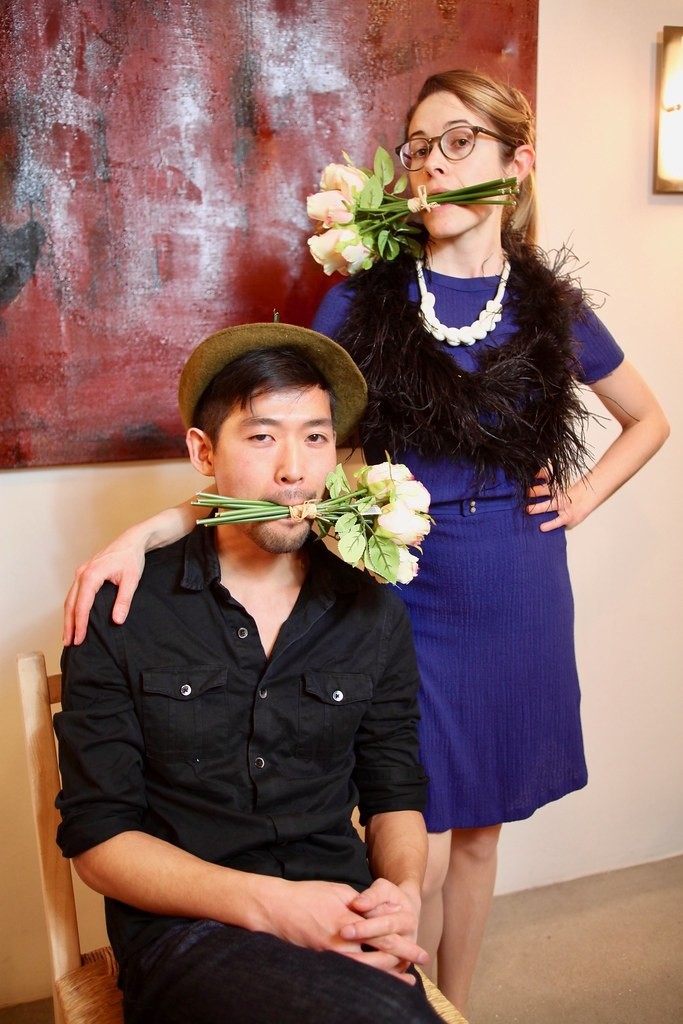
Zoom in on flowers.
[307,146,520,277]
[191,451,436,588]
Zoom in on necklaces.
[416,248,512,346]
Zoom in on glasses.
[395,126,519,171]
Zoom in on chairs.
[16,651,470,1024]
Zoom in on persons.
[48,322,446,1023]
[69,70,670,1011]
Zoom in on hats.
[177,323,368,446]
